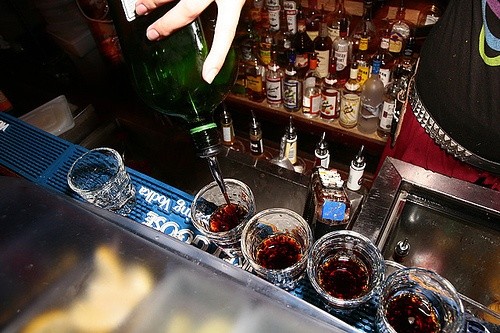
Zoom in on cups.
[190,180,257,259]
[240,206,313,285]
[306,230,386,314]
[67,147,136,211]
[375,265,466,333]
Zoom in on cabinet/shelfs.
[216,0,430,195]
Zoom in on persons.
[135,0,500,197]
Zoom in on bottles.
[279,116,298,166]
[201,0,442,139]
[248,110,264,157]
[346,145,367,190]
[313,131,330,170]
[105,0,238,158]
[220,107,236,145]
[75,0,123,65]
